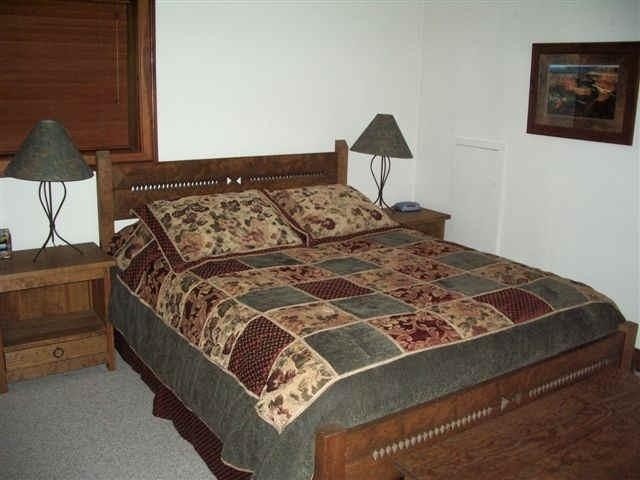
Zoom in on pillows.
[128,183,400,269]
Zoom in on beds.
[96,140,639,480]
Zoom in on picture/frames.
[526,39,640,146]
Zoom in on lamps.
[5,121,93,263]
[347,113,412,215]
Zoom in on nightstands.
[1,242,118,396]
[380,203,450,240]
